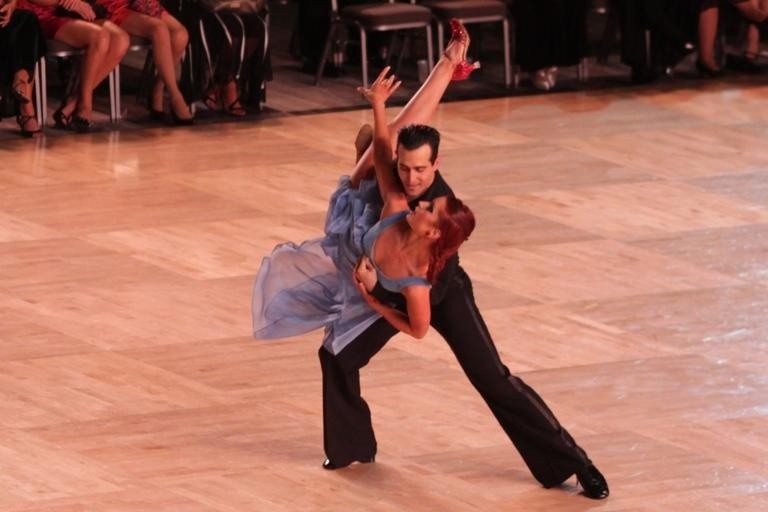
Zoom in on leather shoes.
[575,461,609,500]
[323,446,377,470]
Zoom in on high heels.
[443,18,482,82]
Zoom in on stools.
[314,0,510,101]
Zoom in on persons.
[1,1,269,139]
[322,16,482,341]
[313,121,611,500]
[292,0,346,76]
[504,1,768,90]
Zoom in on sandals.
[16,92,247,138]
